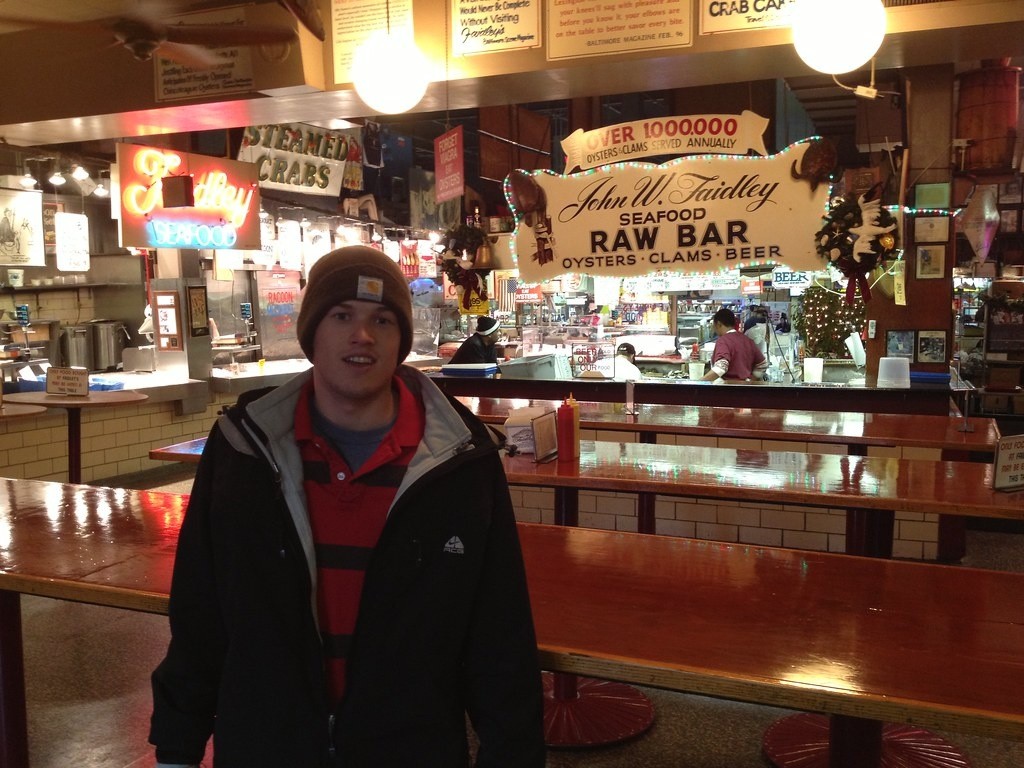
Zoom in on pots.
[58,322,95,372]
[80,318,130,370]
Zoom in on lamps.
[351,1,428,113]
[789,0,887,76]
[19,152,108,197]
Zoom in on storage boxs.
[442,354,556,376]
[985,365,1024,416]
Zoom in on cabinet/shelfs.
[953,274,1024,415]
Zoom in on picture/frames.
[975,180,1024,237]
[915,184,950,279]
[885,329,947,366]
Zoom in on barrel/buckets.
[7,268,24,287]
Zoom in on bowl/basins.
[31,274,87,285]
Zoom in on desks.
[0,391,1024,768]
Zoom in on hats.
[617,343,636,366]
[297,246,413,366]
[476,316,500,336]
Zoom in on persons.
[999,181,1021,203]
[699,308,768,382]
[448,316,501,364]
[208,310,220,348]
[775,317,790,333]
[147,245,547,768]
[591,343,643,381]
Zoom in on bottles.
[693,342,699,361]
[557,398,580,461]
